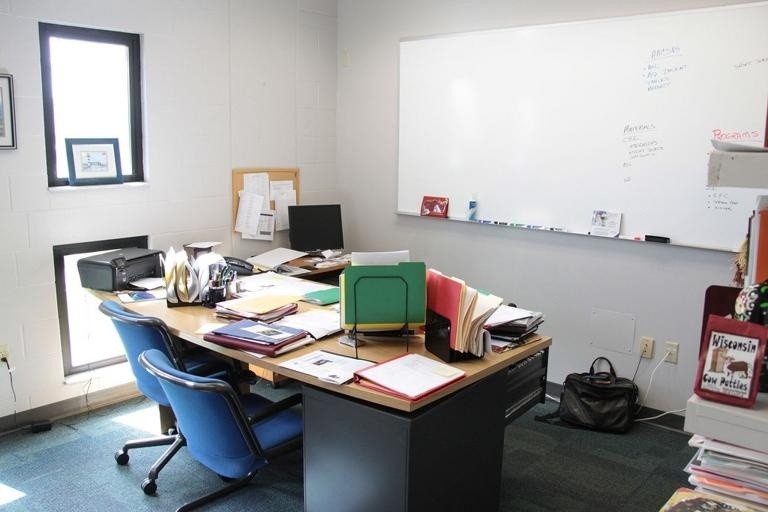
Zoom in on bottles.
[468,200,478,221]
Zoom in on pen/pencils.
[212,264,232,287]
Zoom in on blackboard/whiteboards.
[394,0,768,253]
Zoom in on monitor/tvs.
[288,204,344,257]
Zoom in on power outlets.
[1,342,10,366]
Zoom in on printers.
[78,247,166,291]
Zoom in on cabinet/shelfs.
[683,144,767,453]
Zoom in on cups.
[210,281,238,304]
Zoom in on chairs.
[98,298,256,498]
[135,347,302,510]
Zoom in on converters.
[31,421,51,433]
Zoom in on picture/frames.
[64,137,123,186]
[0,73,18,150]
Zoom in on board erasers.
[645,235,670,243]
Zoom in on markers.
[618,235,641,241]
[478,220,562,232]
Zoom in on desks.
[89,268,552,510]
[281,247,349,280]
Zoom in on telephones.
[224,257,254,275]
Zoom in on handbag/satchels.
[558,356,639,436]
